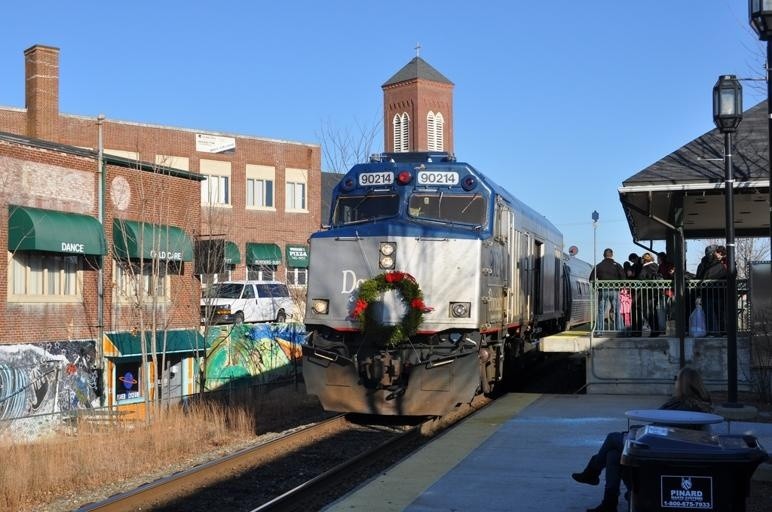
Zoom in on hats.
[705,246,715,254]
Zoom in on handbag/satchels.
[666,320,676,337]
[642,319,651,337]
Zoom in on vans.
[200,280,295,326]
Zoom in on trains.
[302,151,593,417]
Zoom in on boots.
[587,485,620,511]
[572,455,606,484]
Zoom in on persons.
[570,364,712,512]
[587,244,739,337]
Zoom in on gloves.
[696,298,702,306]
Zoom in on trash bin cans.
[620,424,767,512]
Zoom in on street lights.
[710,76,748,410]
[590,208,599,281]
[746,1,771,279]
